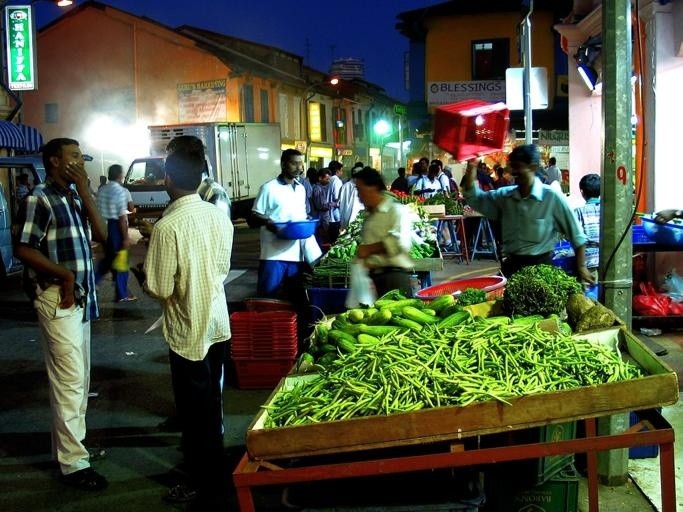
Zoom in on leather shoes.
[50,465,109,493]
[170,480,198,502]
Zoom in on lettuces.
[454,285,487,309]
[500,265,583,315]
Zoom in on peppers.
[325,220,362,265]
[408,240,439,261]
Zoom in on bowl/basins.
[273,219,319,240]
[640,216,683,244]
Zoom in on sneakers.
[445,239,460,247]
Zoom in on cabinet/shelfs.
[315,228,445,292]
[232,281,678,512]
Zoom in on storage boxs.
[229,309,298,391]
[308,287,350,320]
[435,97,509,162]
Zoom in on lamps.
[573,42,601,91]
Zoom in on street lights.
[298,76,339,155]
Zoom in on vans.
[0,156,88,285]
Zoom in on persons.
[654,208,683,224]
[124,188,134,212]
[494,167,510,187]
[407,162,419,186]
[251,148,306,307]
[414,164,442,200]
[418,157,430,179]
[431,160,451,199]
[17,173,31,209]
[137,135,231,243]
[354,166,414,302]
[140,150,232,503]
[478,163,494,191]
[300,167,318,216]
[97,176,107,191]
[574,173,601,286]
[313,169,340,244]
[16,137,110,493]
[390,167,409,194]
[338,167,365,229]
[93,164,140,304]
[460,143,597,288]
[545,157,562,184]
[325,159,343,242]
[443,169,458,197]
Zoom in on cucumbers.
[297,290,571,372]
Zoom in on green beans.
[260,315,644,432]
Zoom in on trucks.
[120,121,282,235]
